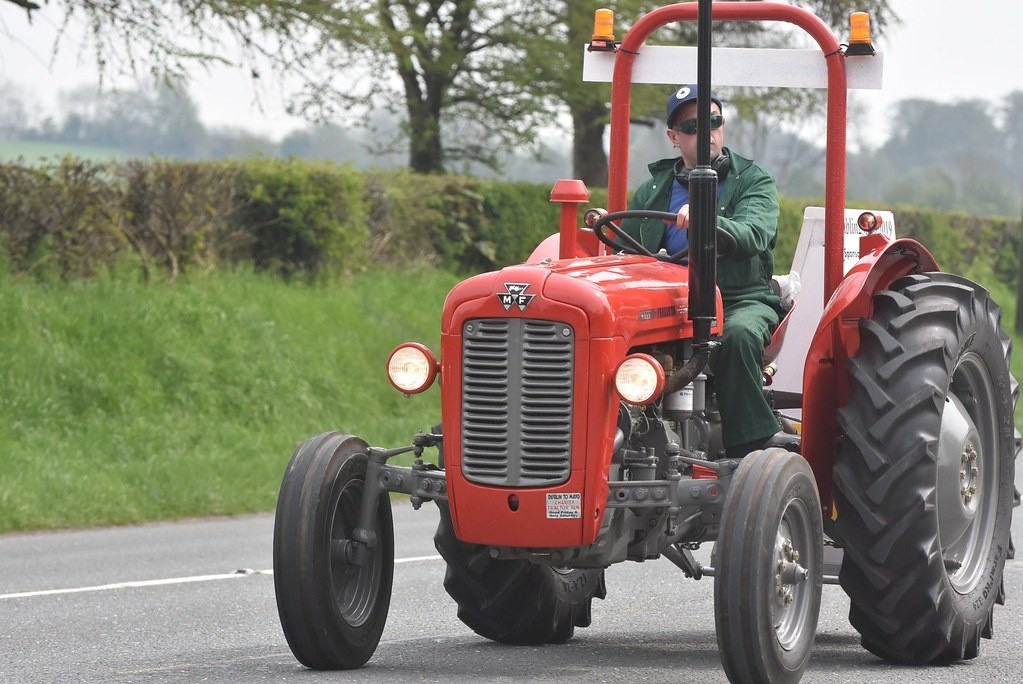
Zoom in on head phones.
[673,147,729,189]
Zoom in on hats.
[666,84,722,129]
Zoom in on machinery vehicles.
[273,1,1023,683]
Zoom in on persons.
[613,83,782,458]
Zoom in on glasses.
[672,115,723,134]
